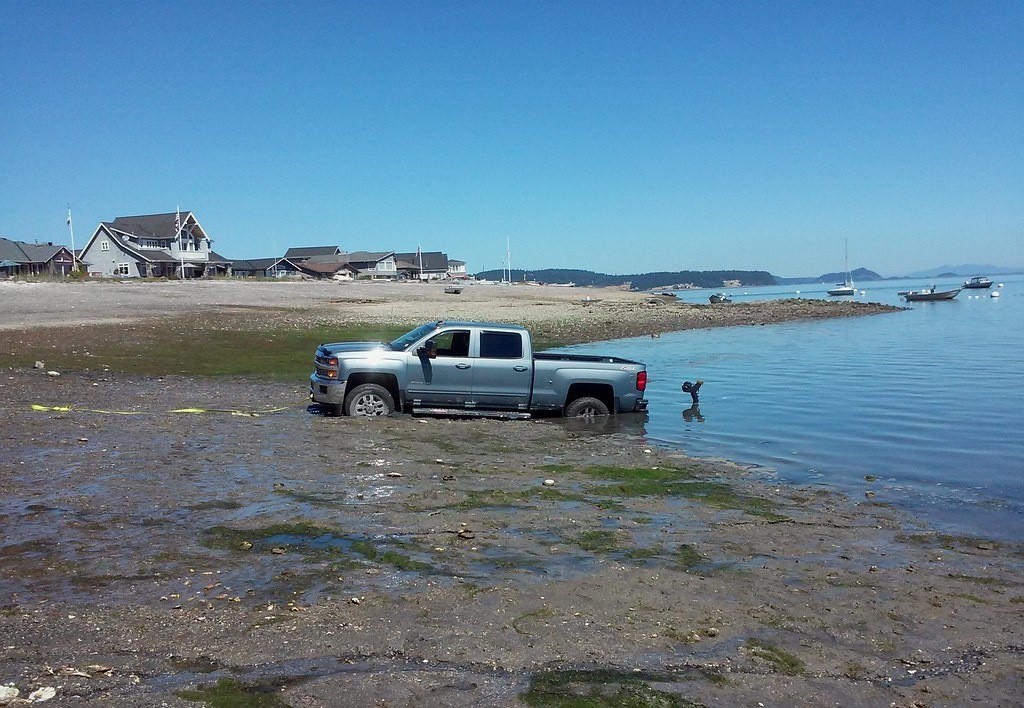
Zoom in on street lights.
[502,261,506,281]
[67,209,76,272]
[174,205,185,278]
[507,238,511,283]
[415,244,423,283]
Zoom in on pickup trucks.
[310,321,649,419]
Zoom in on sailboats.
[827,236,857,295]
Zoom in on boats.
[730,293,732,297]
[709,293,732,303]
[445,285,464,294]
[860,291,865,295]
[961,276,993,288]
[743,291,748,296]
[581,295,603,302]
[897,284,965,303]
[795,289,801,294]
